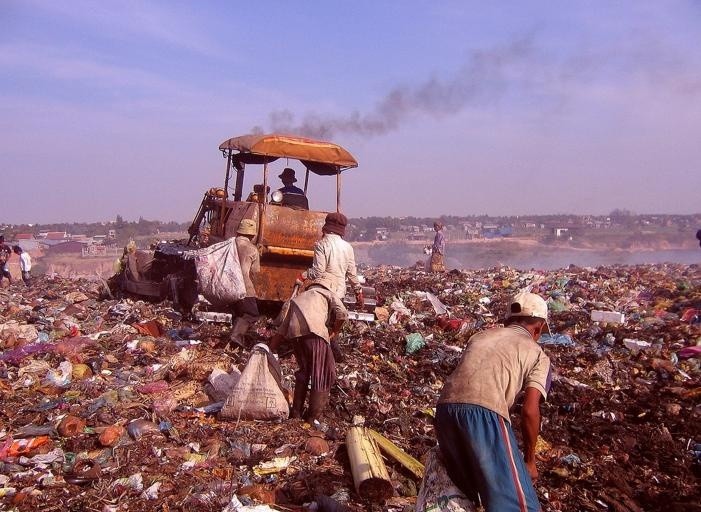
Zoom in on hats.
[237,218,257,236]
[508,292,552,338]
[322,212,348,236]
[278,168,297,182]
[305,278,336,293]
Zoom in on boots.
[291,379,329,425]
[330,339,344,362]
[230,316,249,350]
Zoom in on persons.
[272,168,308,209]
[223,218,260,330]
[435,292,551,512]
[0,235,32,287]
[271,211,365,423]
[431,221,445,273]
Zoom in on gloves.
[355,293,364,311]
[291,270,307,288]
[270,336,281,354]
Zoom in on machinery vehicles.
[186,131,361,305]
